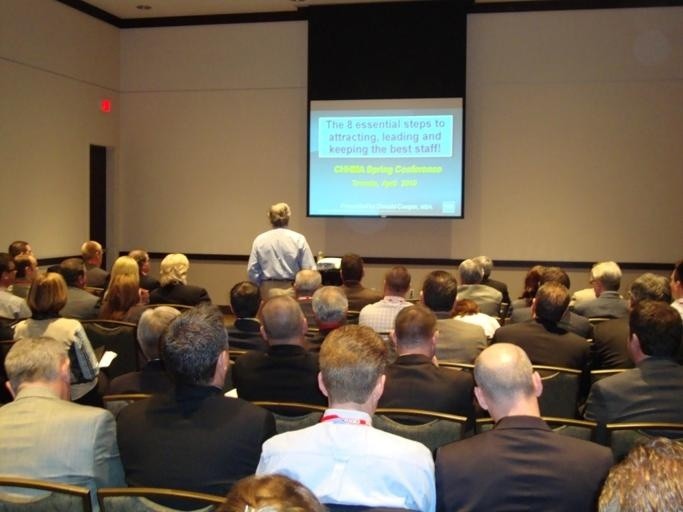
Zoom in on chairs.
[96,486,227,511]
[606,423,683,444]
[370,410,467,458]
[477,417,598,447]
[530,364,583,416]
[0,476,92,511]
[248,400,347,440]
[85,320,138,376]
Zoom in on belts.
[267,278,293,282]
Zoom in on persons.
[9,240,33,257]
[81,240,110,288]
[593,271,671,369]
[511,266,546,308]
[359,265,415,333]
[420,271,486,367]
[13,255,39,296]
[489,281,590,404]
[247,202,317,302]
[308,285,349,363]
[0,252,32,319]
[292,269,322,303]
[116,306,277,511]
[225,281,269,351]
[99,275,142,322]
[512,267,594,340]
[669,260,683,320]
[104,255,147,304]
[216,474,330,512]
[451,299,501,339]
[231,296,329,417]
[255,325,436,512]
[127,249,161,291]
[108,306,181,394]
[59,258,100,321]
[574,260,631,318]
[338,253,383,311]
[377,305,476,432]
[2,337,129,511]
[570,268,597,310]
[581,300,683,439]
[598,436,683,512]
[436,343,613,511]
[473,256,511,305]
[13,272,107,397]
[456,259,502,317]
[150,253,212,308]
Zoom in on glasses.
[589,280,596,284]
[627,289,636,300]
[9,266,18,272]
